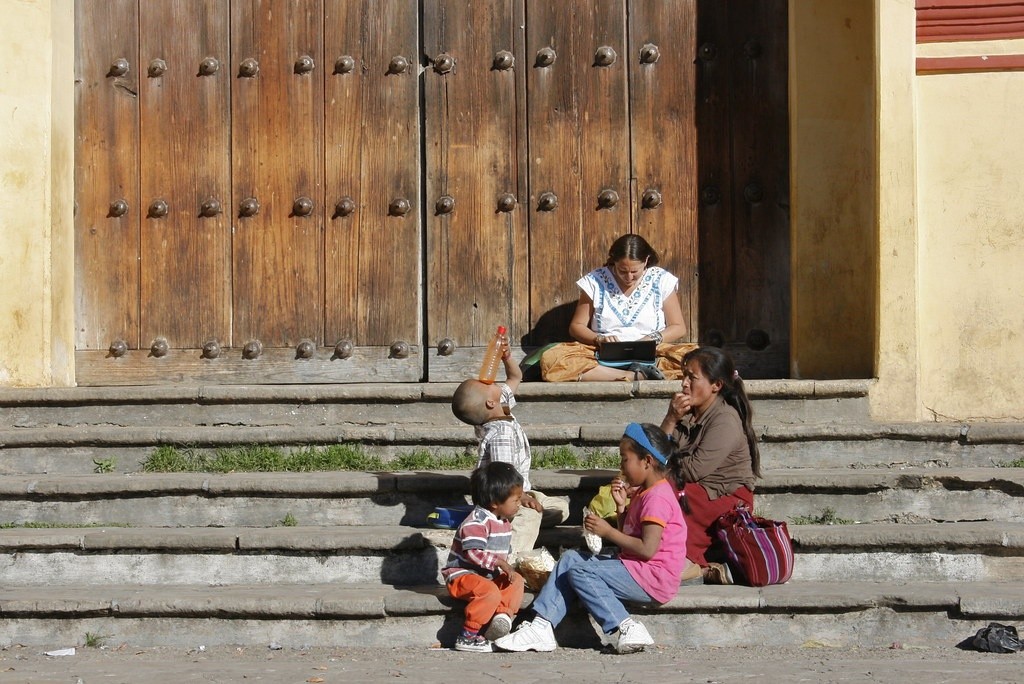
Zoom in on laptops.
[600,340,656,362]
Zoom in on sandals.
[628,362,665,381]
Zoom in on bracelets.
[647,331,663,345]
[595,333,605,346]
[614,506,627,514]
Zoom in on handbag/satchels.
[717,507,795,587]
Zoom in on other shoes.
[455,635,499,653]
[708,563,733,585]
[485,612,512,641]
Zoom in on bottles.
[480,325,507,385]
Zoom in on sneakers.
[617,621,654,654]
[494,619,557,651]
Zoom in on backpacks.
[426,503,475,530]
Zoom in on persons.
[659,346,762,581]
[451,334,569,570]
[441,461,524,652]
[540,234,702,382]
[494,421,688,653]
